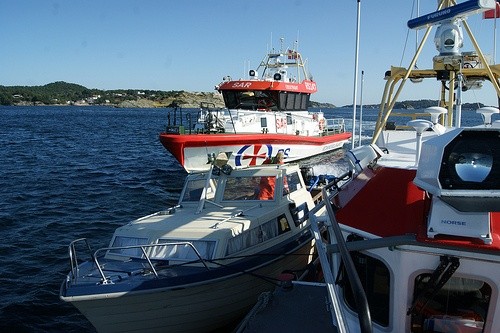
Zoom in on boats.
[59,142,377,333]
[234,0,500,332]
[159,30,352,173]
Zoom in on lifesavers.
[318,118,325,130]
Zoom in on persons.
[259,154,289,201]
